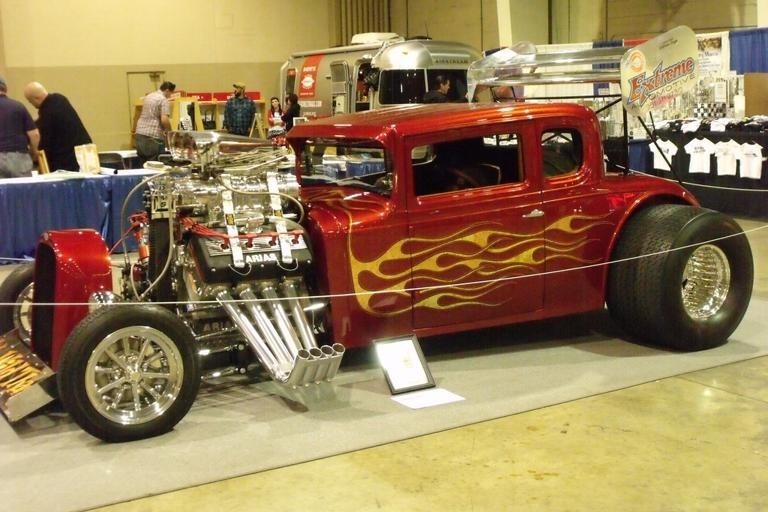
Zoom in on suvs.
[1,97,755,447]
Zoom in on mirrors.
[373,333,435,395]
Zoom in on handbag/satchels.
[202,110,215,129]
[268,126,287,138]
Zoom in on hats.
[233,82,247,89]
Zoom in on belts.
[7,149,28,153]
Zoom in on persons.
[24,82,92,171]
[266,97,287,138]
[1,77,40,177]
[135,81,176,169]
[281,94,301,133]
[421,75,451,102]
[224,82,256,136]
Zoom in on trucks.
[274,26,487,121]
